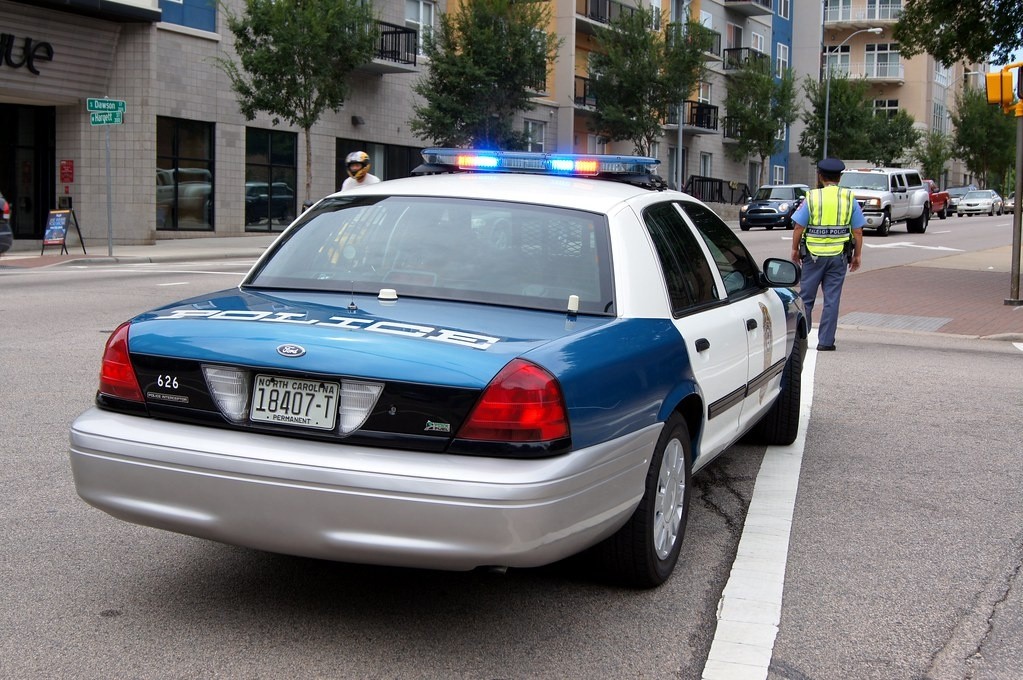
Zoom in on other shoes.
[816,344,837,351]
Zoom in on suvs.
[837,167,931,236]
[156,166,213,228]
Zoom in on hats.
[818,157,845,175]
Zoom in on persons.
[342,151,381,190]
[791,158,867,351]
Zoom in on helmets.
[345,150,372,181]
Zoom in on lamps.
[351,116,365,126]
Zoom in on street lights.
[941,71,978,139]
[823,28,884,161]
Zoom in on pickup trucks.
[921,179,951,219]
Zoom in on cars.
[69,145,810,593]
[943,185,1016,216]
[0,193,14,252]
[739,183,811,231]
[245,181,296,224]
